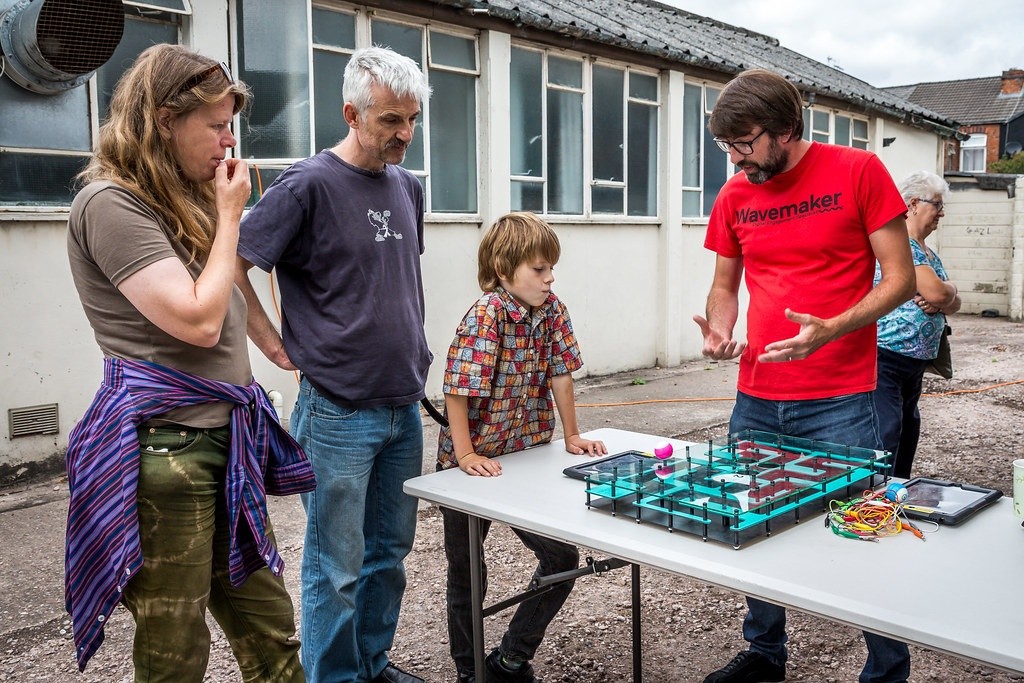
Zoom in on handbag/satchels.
[923,314,952,379]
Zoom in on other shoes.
[484,649,537,683]
[374,661,426,683]
[457,674,475,683]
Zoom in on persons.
[230,46,438,681]
[872,171,963,482]
[435,212,609,683]
[694,71,918,683]
[65,43,307,683]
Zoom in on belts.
[421,397,449,428]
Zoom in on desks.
[401,427,1023,683]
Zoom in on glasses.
[174,61,233,97]
[712,128,768,156]
[908,199,944,212]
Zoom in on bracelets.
[938,308,943,312]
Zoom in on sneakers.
[704,650,786,683]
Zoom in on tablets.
[881,476,1002,525]
[563,450,678,486]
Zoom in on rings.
[788,356,792,362]
[923,301,925,305]
[925,305,927,309]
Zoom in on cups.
[1013,459,1024,519]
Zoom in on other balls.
[885,482,908,504]
[654,441,674,459]
